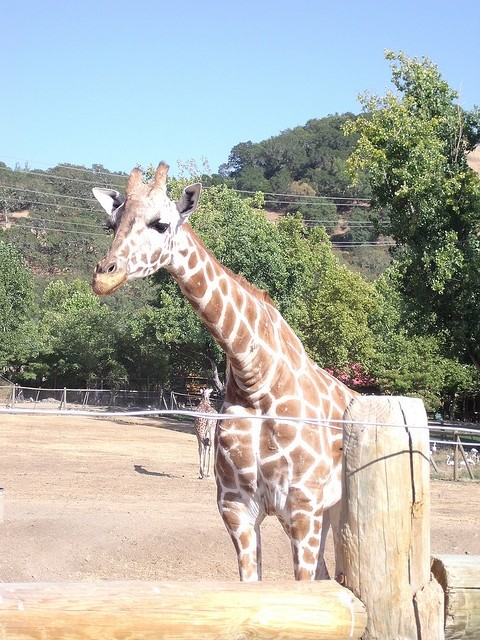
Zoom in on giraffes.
[87,159,360,578]
[193,387,219,479]
